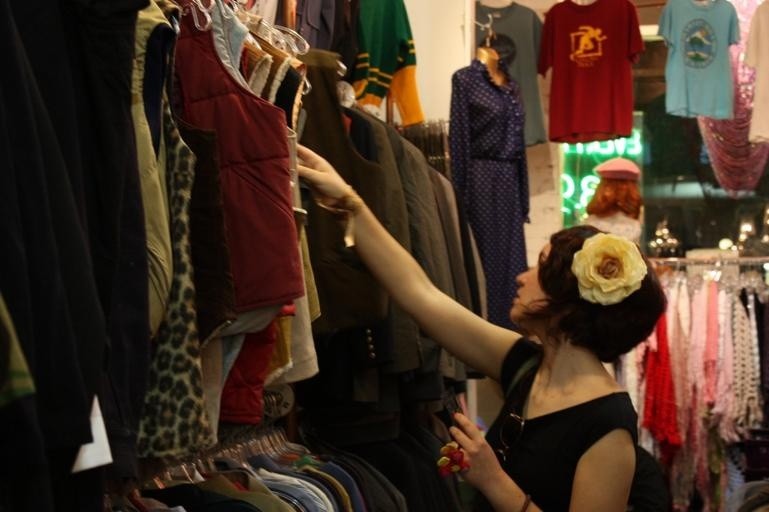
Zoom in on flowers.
[571,232,650,307]
[435,446,470,483]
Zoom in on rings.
[456,444,462,451]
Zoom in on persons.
[448,45,531,327]
[296,144,668,512]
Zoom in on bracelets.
[520,493,531,512]
[330,188,364,221]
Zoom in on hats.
[591,156,642,184]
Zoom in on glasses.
[494,410,524,475]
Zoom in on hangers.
[655,255,769,301]
[155,0,348,96]
[98,391,324,512]
[423,118,450,167]
